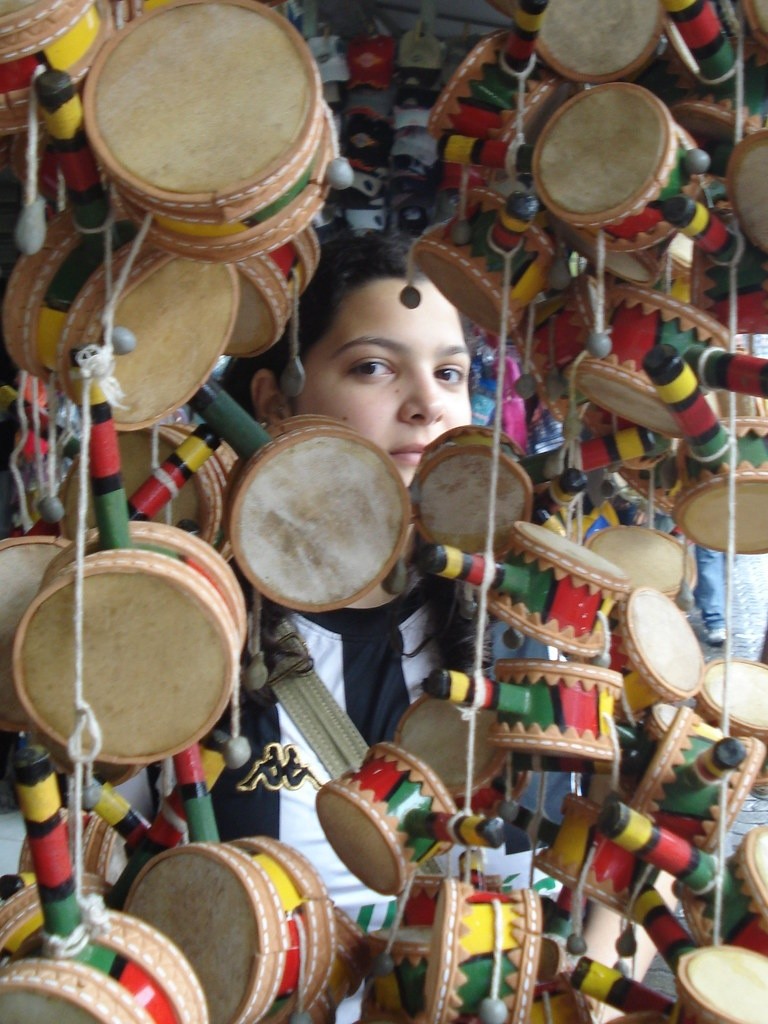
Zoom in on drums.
[0,2,767,1022]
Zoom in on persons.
[653,512,727,645]
[66,229,680,1024]
